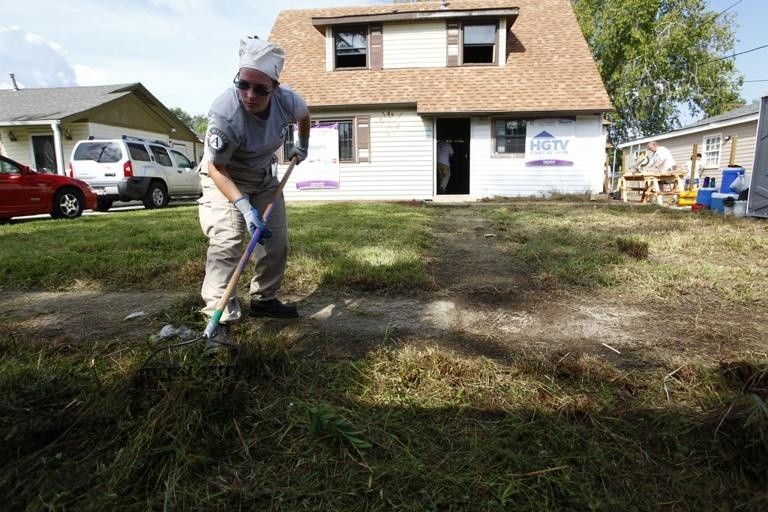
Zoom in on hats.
[238,35,286,81]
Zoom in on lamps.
[724,136,730,140]
[63,130,72,140]
[7,131,17,142]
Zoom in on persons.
[644,138,677,178]
[194,40,311,359]
[685,152,704,188]
[436,135,454,195]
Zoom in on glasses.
[233,72,279,96]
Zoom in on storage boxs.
[696,167,748,216]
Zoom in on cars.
[0,155,98,218]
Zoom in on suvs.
[70,135,203,212]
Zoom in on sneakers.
[247,298,300,319]
[204,321,229,357]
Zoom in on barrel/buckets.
[696,187,719,208]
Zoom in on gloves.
[234,193,273,246]
[288,134,309,165]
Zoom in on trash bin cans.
[695,188,741,212]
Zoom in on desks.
[618,169,685,205]
